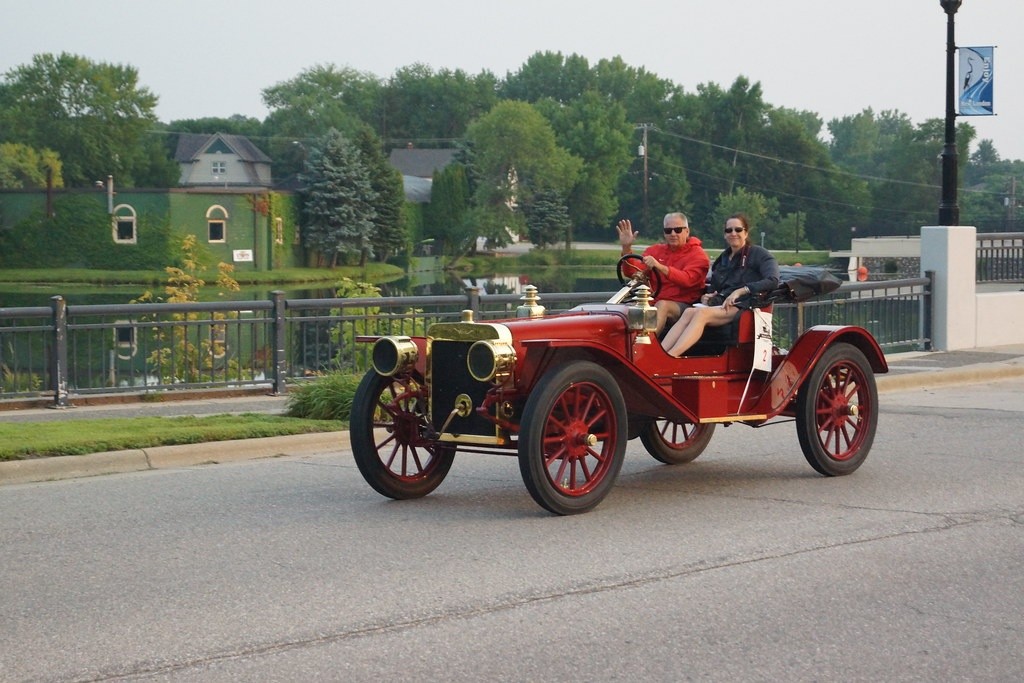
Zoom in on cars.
[349,254,889,516]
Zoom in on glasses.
[663,227,686,234]
[724,227,746,234]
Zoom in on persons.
[616,212,710,338]
[661,214,779,356]
